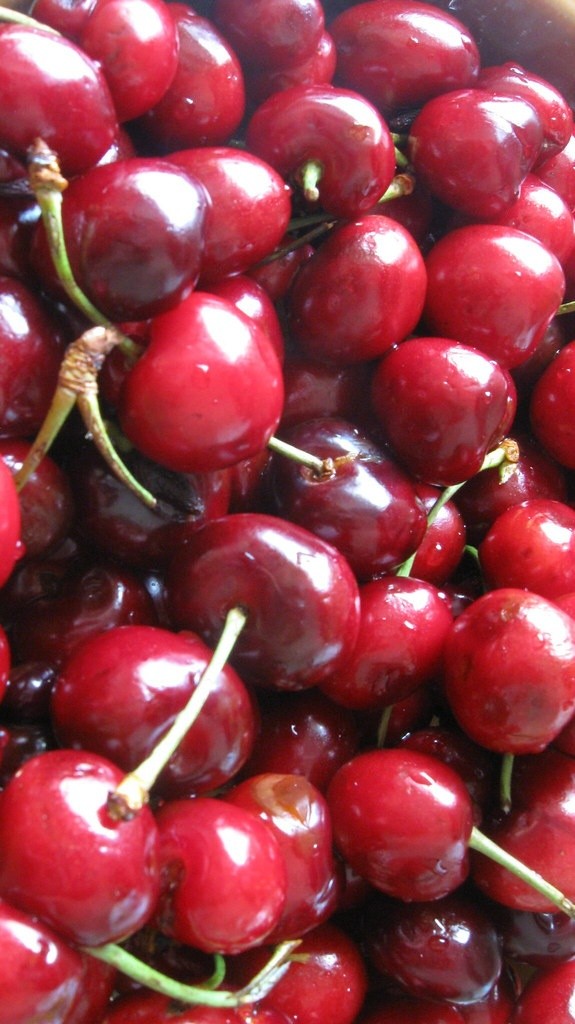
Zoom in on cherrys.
[0,0,575,1024]
